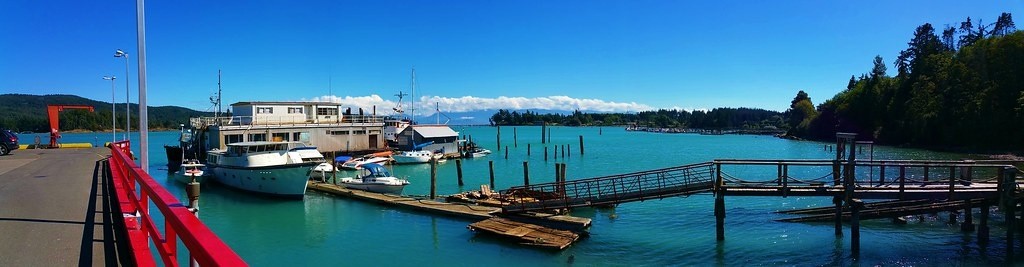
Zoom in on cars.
[0,128,20,156]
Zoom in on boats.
[164,69,327,197]
[314,68,492,194]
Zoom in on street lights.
[114,49,130,142]
[102,76,115,143]
